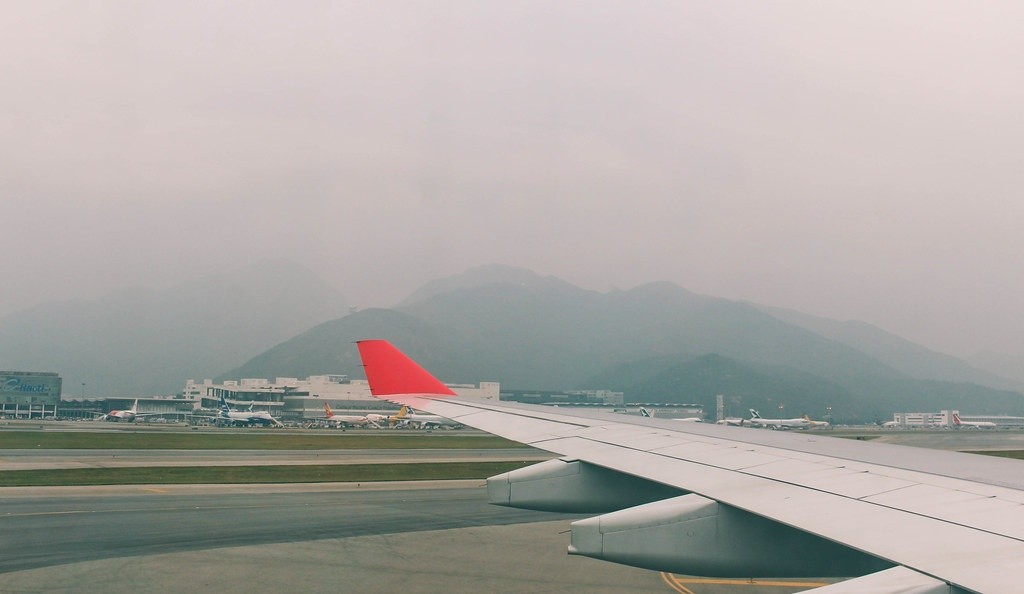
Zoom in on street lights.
[81,383,86,418]
[778,405,784,426]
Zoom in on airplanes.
[356,339,1024,593]
[717,411,759,427]
[749,408,830,430]
[386,406,464,429]
[316,402,367,429]
[639,407,701,423]
[105,399,138,422]
[952,414,997,429]
[215,394,272,427]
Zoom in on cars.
[1005,426,1009,429]
[149,418,157,423]
[935,427,940,430]
[930,427,935,430]
[775,424,791,431]
[944,426,948,430]
[311,423,318,428]
[396,424,403,429]
[167,419,178,424]
[157,418,166,424]
[1019,426,1023,429]
[33,416,94,422]
[1000,426,1004,429]
